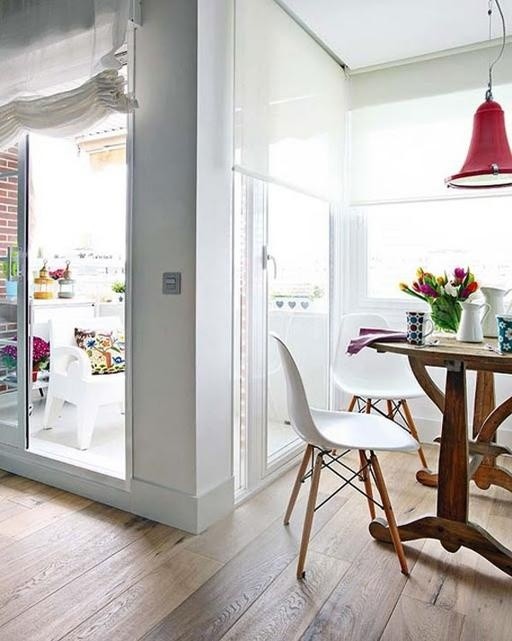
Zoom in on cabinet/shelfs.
[31,301,97,398]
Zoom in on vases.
[3,333,50,372]
[33,372,38,383]
[399,266,478,334]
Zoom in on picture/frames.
[362,331,511,586]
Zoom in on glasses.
[443,0,512,191]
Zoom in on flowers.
[399,266,478,334]
[3,333,50,372]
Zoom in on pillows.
[73,327,124,375]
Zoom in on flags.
[33,372,38,383]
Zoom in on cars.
[406,284,512,354]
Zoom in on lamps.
[443,0,512,191]
[42,318,125,451]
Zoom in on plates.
[31,301,97,398]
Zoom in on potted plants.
[113,280,128,304]
[0,241,20,301]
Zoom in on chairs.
[42,318,125,451]
[333,312,428,481]
[272,336,420,580]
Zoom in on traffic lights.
[73,327,124,375]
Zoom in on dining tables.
[113,280,128,304]
[0,241,20,301]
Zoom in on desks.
[362,331,511,586]
